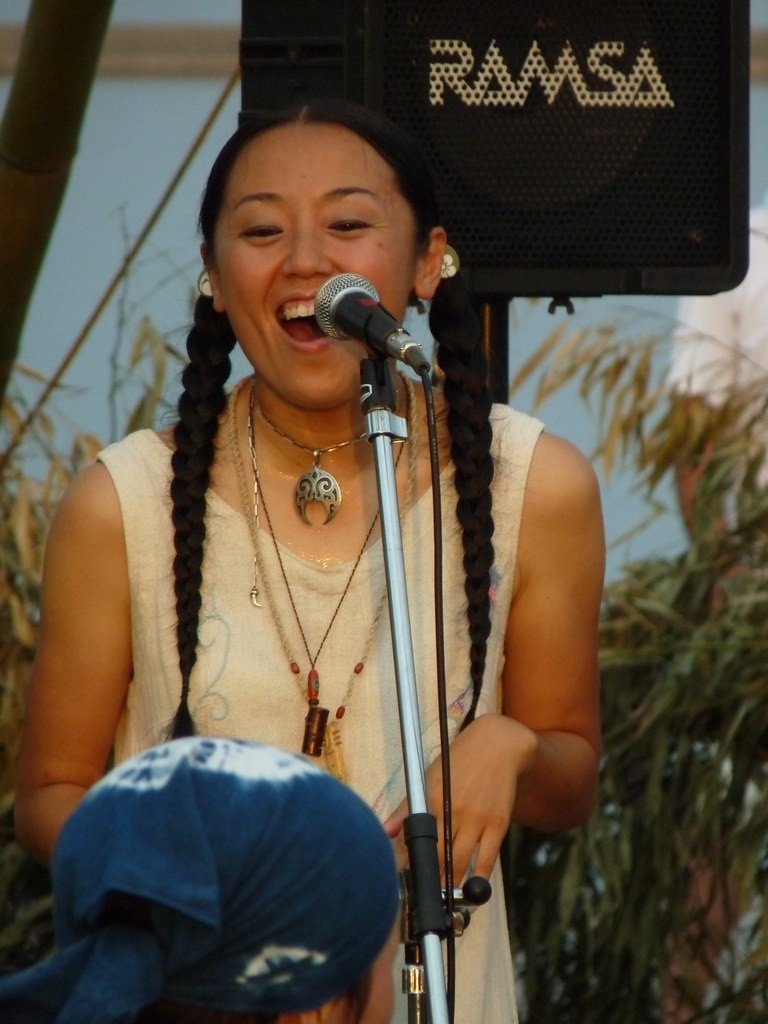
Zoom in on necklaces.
[225,372,418,783]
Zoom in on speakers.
[239,0,751,301]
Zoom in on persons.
[0,736,403,1024]
[0,85,604,1024]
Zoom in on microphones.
[315,273,432,376]
